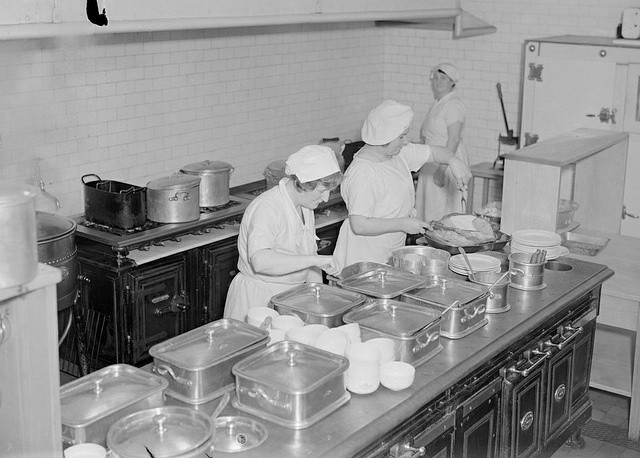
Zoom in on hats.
[285,145,340,183]
[360,99,413,146]
[431,63,460,82]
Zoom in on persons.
[413,62,470,219]
[333,98,473,271]
[222,144,349,322]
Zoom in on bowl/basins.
[246,305,416,394]
[230,339,351,431]
[107,406,215,457]
[423,228,511,255]
[325,260,429,301]
[558,200,578,228]
[59,363,169,451]
[398,275,491,340]
[341,299,444,367]
[64,441,105,458]
[390,244,452,273]
[148,317,271,406]
[270,280,367,328]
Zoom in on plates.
[448,251,501,277]
[509,231,562,261]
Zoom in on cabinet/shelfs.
[501,338,572,457]
[570,314,597,417]
[124,254,189,370]
[413,410,455,458]
[458,376,502,458]
[199,241,240,323]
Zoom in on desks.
[466,162,503,215]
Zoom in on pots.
[1,187,38,289]
[264,159,289,191]
[147,174,202,223]
[36,210,80,313]
[180,159,235,209]
[81,173,148,230]
